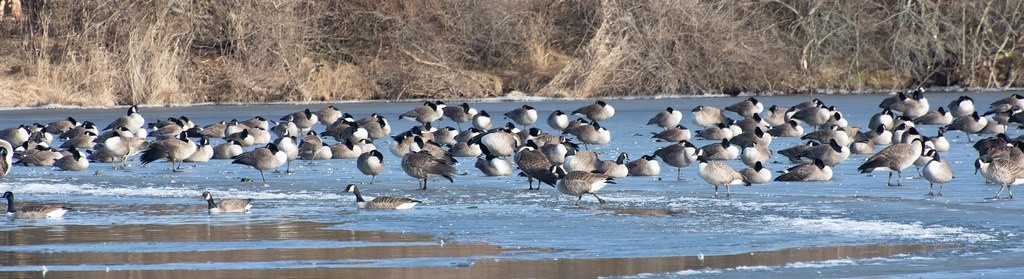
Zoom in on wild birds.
[341,184,422,211]
[390,98,660,206]
[645,87,1024,201]
[1,103,391,184]
[201,190,253,215]
[1,192,71,220]
